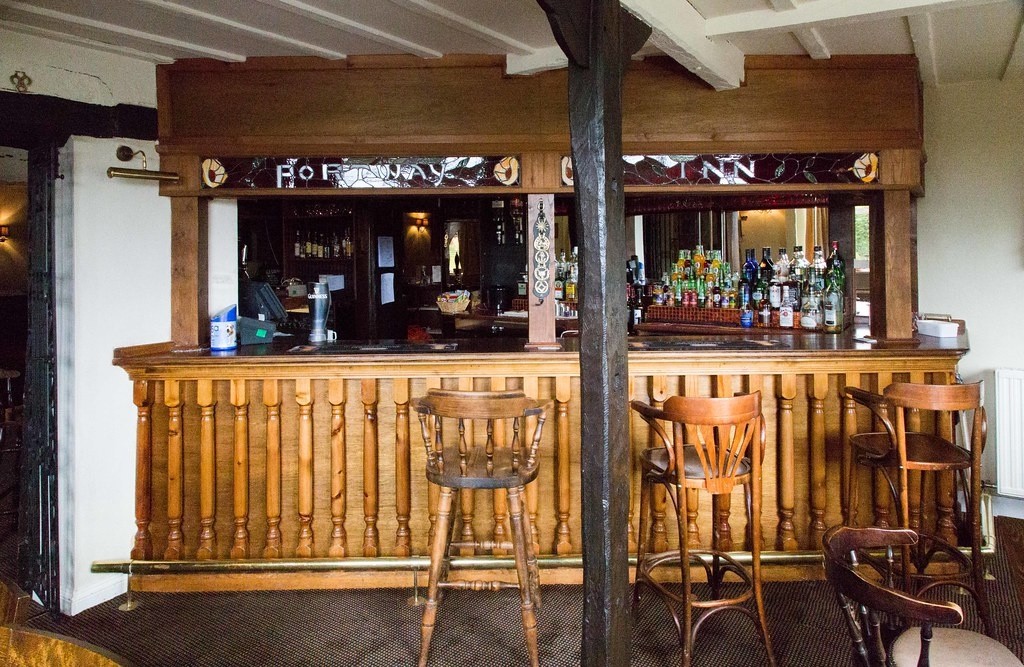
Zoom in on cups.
[308,282,330,342]
[326,330,337,341]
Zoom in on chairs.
[819,522,1024,667]
[412,387,554,667]
[843,380,990,598]
[629,390,779,667]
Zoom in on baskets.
[436,300,471,313]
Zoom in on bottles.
[626,241,846,334]
[294,227,353,259]
[554,247,578,302]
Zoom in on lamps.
[106,145,181,185]
